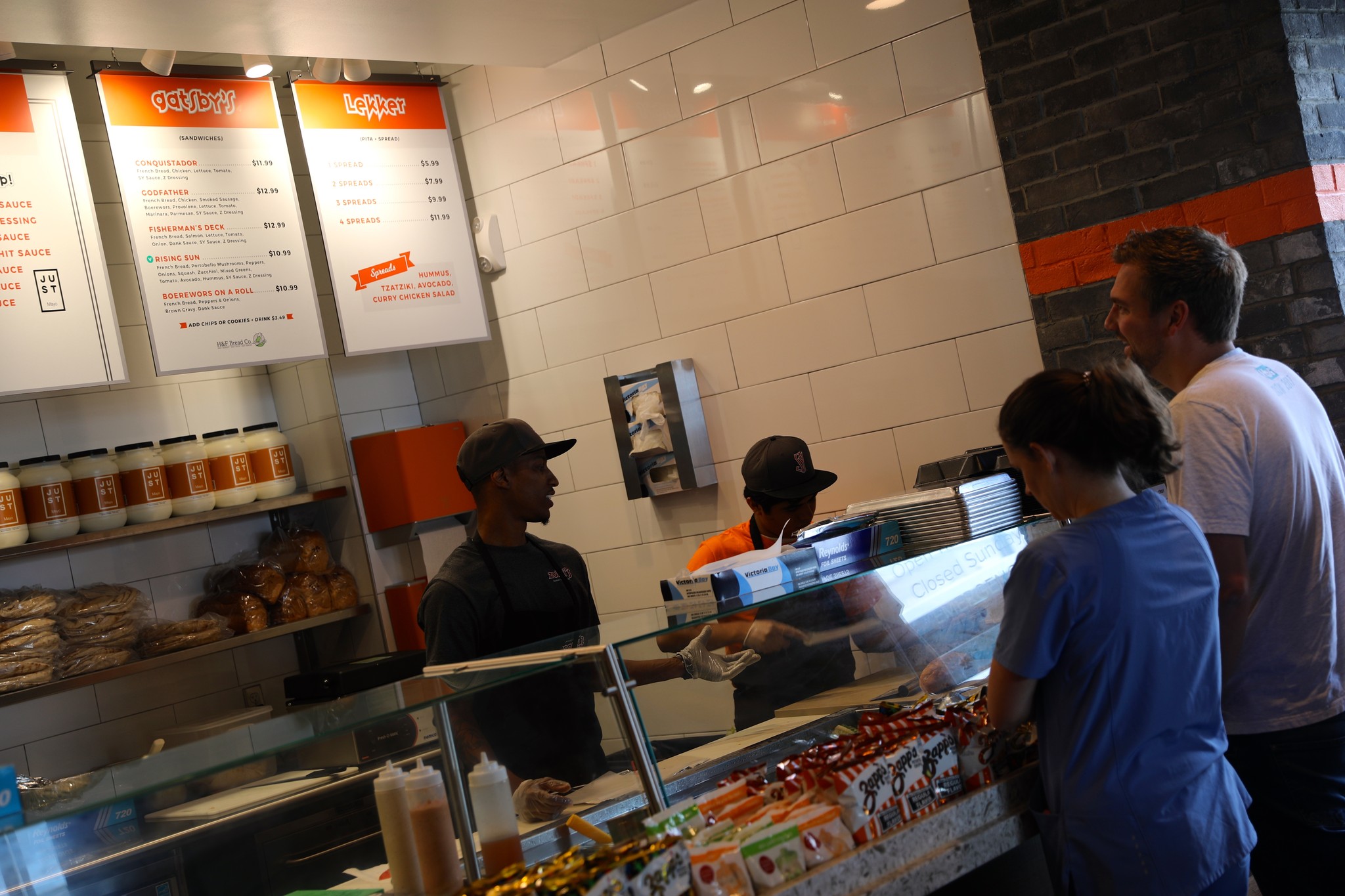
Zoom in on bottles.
[373,760,426,896]
[405,758,466,896]
[468,752,526,877]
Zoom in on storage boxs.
[0,762,138,853]
[153,704,275,795]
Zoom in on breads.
[199,532,360,634]
[919,652,979,694]
[0,583,226,692]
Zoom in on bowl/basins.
[92,754,187,816]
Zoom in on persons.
[418,419,760,824]
[1103,225,1345,896]
[654,435,921,732]
[986,355,1266,896]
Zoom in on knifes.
[240,767,347,789]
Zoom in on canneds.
[0,421,297,550]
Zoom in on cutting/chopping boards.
[775,659,993,718]
[144,767,359,820]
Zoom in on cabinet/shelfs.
[0,484,372,708]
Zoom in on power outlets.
[243,684,265,708]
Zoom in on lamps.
[0,42,16,62]
[344,59,372,82]
[242,54,274,78]
[139,49,176,76]
[312,58,342,83]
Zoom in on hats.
[456,418,577,491]
[741,435,838,499]
